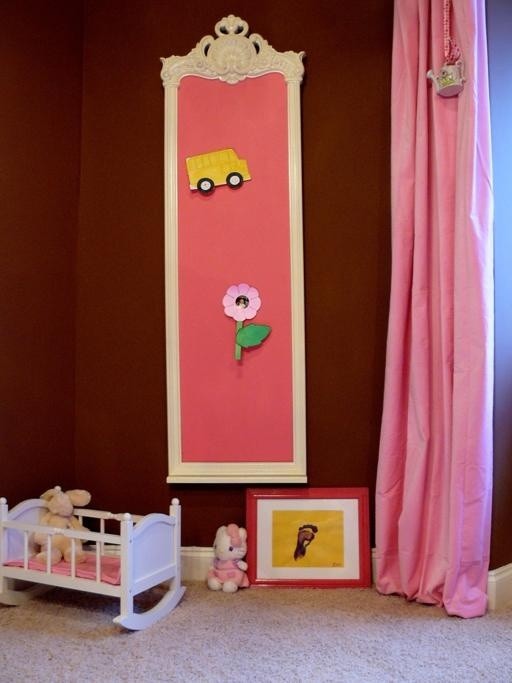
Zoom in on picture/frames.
[163,10,314,488]
[245,484,375,591]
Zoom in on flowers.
[218,278,273,364]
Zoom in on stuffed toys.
[34,486,92,565]
[207,523,248,592]
